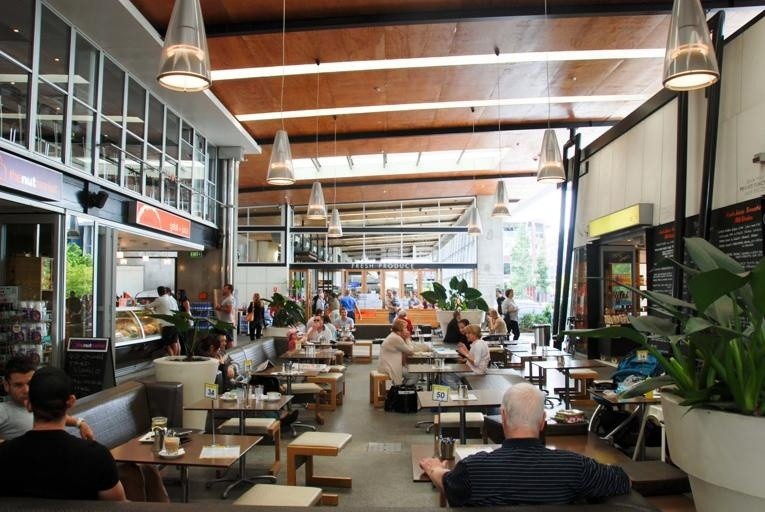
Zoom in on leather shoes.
[281,409,300,428]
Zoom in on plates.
[220,395,237,402]
[158,452,186,459]
[138,430,155,442]
[264,397,284,400]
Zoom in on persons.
[313,289,339,311]
[483,308,508,342]
[146,285,179,356]
[391,291,400,316]
[496,288,506,315]
[210,328,298,428]
[386,289,400,322]
[0,363,131,503]
[306,315,332,343]
[165,286,173,297]
[176,289,194,356]
[340,288,362,324]
[376,319,419,387]
[408,291,422,309]
[305,309,324,330]
[335,308,354,330]
[501,289,520,347]
[198,337,228,391]
[443,311,461,344]
[445,324,491,385]
[396,309,413,335]
[66,291,92,315]
[0,356,94,442]
[247,293,265,341]
[457,319,472,352]
[419,382,632,509]
[214,284,236,350]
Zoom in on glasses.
[313,320,321,321]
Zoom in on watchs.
[429,466,441,478]
[76,417,87,428]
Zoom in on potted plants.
[259,292,306,337]
[556,237,765,512]
[144,309,237,429]
[419,276,490,339]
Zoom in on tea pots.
[151,416,167,438]
[234,380,249,406]
[153,427,171,453]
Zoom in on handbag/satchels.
[504,311,511,330]
[246,302,254,322]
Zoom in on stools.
[307,373,344,411]
[355,341,372,364]
[569,368,596,407]
[328,364,346,405]
[433,412,488,457]
[216,416,281,476]
[369,370,392,408]
[279,383,326,425]
[287,431,352,506]
[232,484,322,507]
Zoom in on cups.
[163,437,182,453]
[254,385,264,404]
[268,392,282,399]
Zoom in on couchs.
[64,379,182,449]
[228,336,354,381]
[0,486,662,511]
[352,325,432,343]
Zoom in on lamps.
[267,0,296,185]
[537,1,566,182]
[91,190,108,208]
[327,115,342,237]
[467,107,483,235]
[306,58,326,221]
[490,48,511,218]
[662,1,721,91]
[155,0,212,93]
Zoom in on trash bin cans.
[530,323,552,348]
